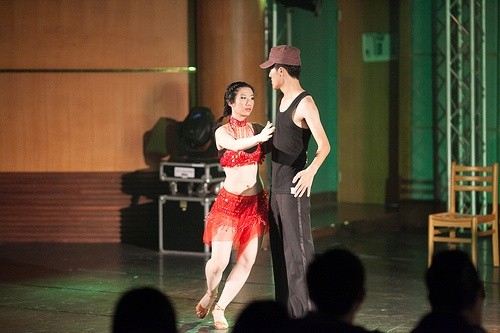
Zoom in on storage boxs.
[159,195,216,256]
[160,162,225,182]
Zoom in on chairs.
[427,162,499,269]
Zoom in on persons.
[112,287,177,333]
[232,246,382,333]
[410,249,488,333]
[195,82,276,329]
[260,45,330,317]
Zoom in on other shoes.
[212,309,230,330]
[196,292,218,319]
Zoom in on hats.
[259,45,302,69]
